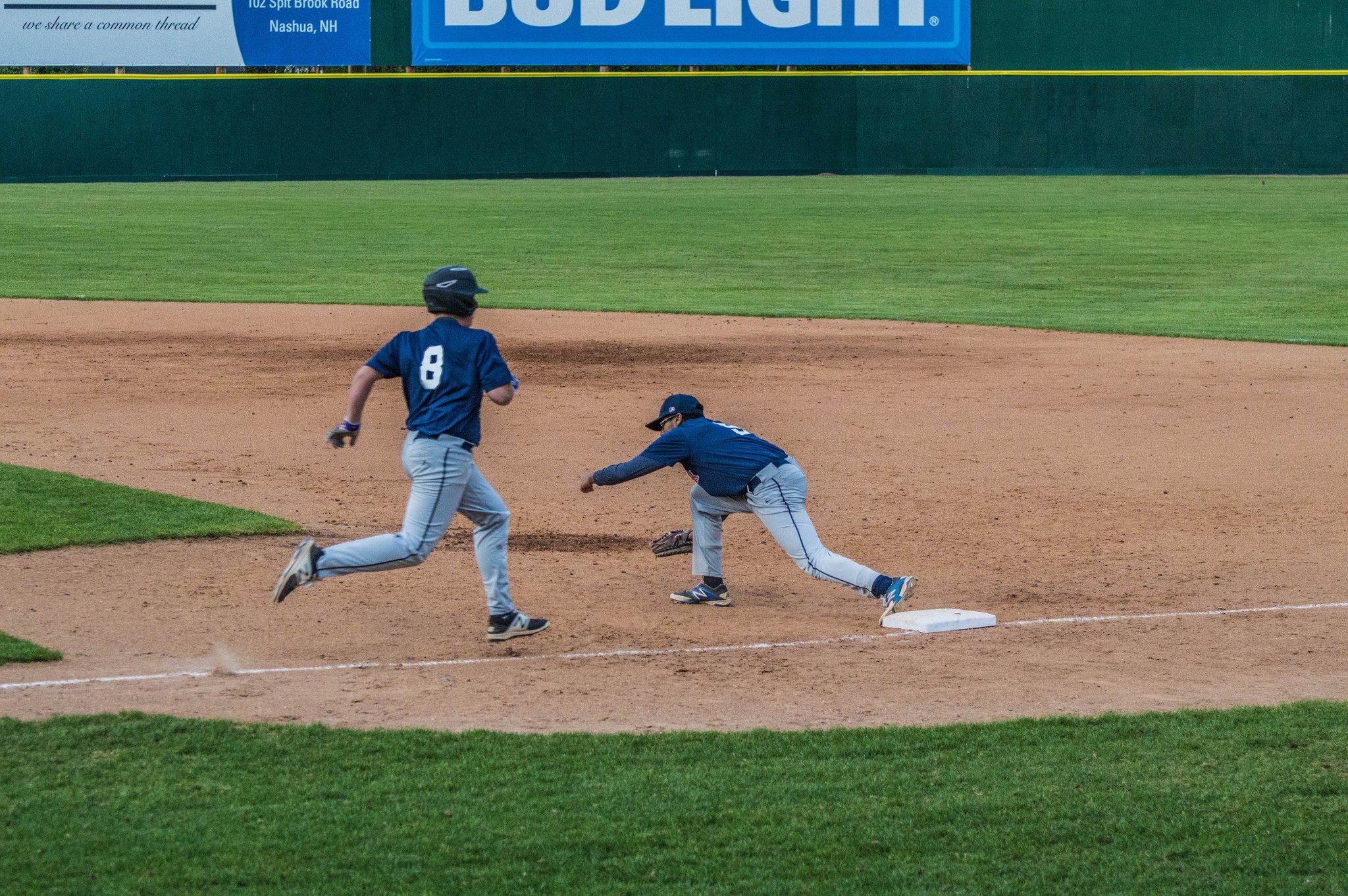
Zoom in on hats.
[646,393,703,432]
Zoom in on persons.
[271,266,550,642]
[578,394,920,628]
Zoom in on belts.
[415,431,473,453]
[750,459,788,491]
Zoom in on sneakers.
[273,537,324,603]
[877,577,917,629]
[486,610,551,641]
[670,575,732,607]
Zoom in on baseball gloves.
[649,529,693,558]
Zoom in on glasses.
[660,412,679,429]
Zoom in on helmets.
[423,265,488,316]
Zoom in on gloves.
[325,418,363,449]
